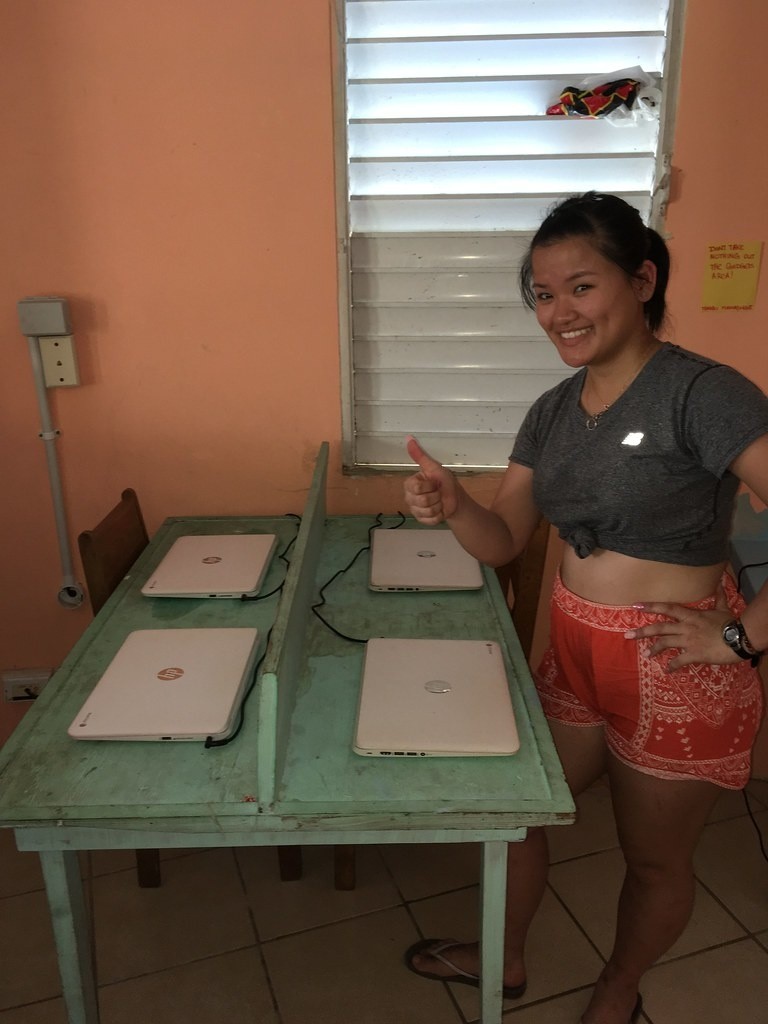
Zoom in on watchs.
[723,620,761,668]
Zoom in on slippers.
[403,936,531,1000]
[578,987,644,1024]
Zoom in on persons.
[405,192,768,1024]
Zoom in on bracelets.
[738,617,763,654]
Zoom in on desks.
[0,440,577,1024]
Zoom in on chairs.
[333,513,551,891]
[79,486,307,888]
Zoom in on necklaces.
[586,341,656,430]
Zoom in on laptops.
[67,628,260,742]
[369,528,485,593]
[141,534,278,598]
[352,638,520,758]
